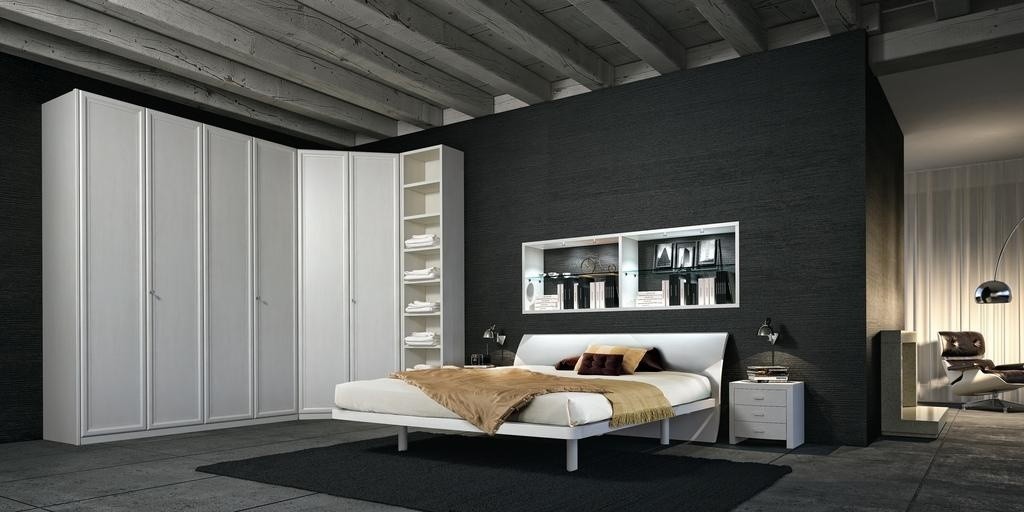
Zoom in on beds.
[331,330,731,472]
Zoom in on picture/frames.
[653,239,720,273]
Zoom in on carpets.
[194,432,791,511]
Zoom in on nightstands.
[728,379,806,450]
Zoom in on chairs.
[937,331,1024,414]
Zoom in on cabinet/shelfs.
[298,150,401,421]
[521,221,740,314]
[400,143,465,372]
[203,122,297,434]
[40,90,203,448]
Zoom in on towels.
[404,234,441,372]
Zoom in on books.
[534,271,736,309]
[746,364,789,383]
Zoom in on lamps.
[975,217,1023,304]
[483,323,496,365]
[757,316,779,364]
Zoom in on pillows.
[554,345,667,377]
